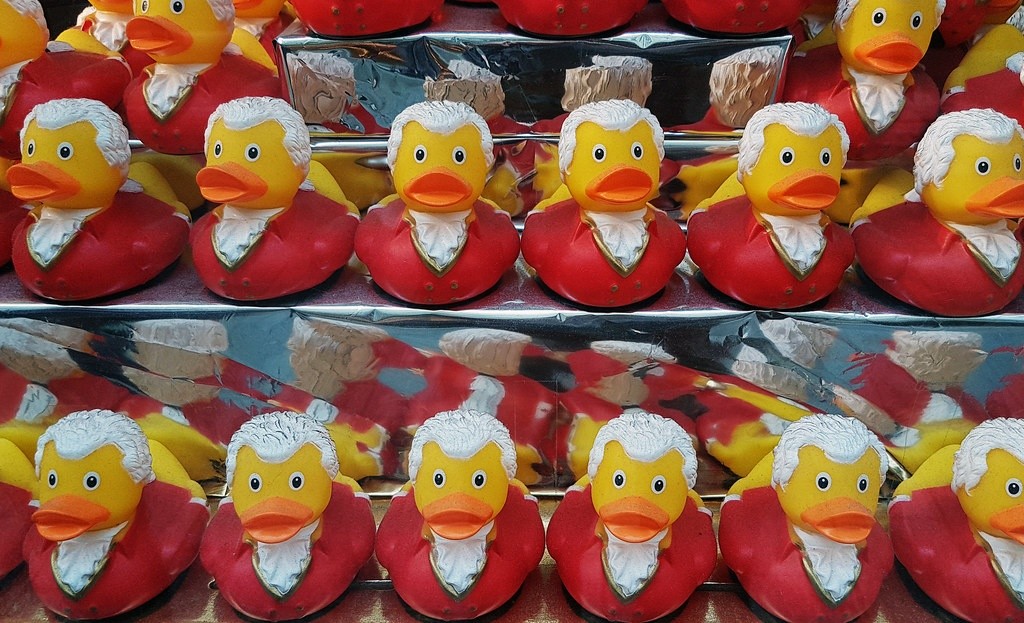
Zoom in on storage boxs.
[272,6,796,141]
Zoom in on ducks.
[1,409,1024,623]
[0,0,1024,316]
